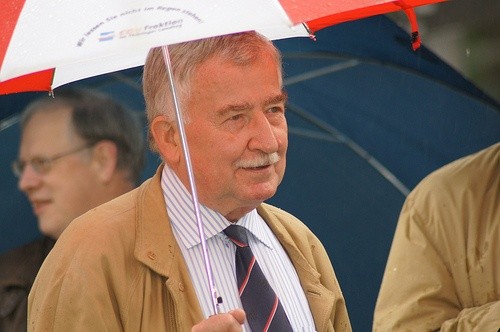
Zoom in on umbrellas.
[0,1,449,313]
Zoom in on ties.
[222,222,293,332]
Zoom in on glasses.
[12,142,99,181]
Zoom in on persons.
[0,93,154,332]
[368,139,499,331]
[26,31,354,332]
